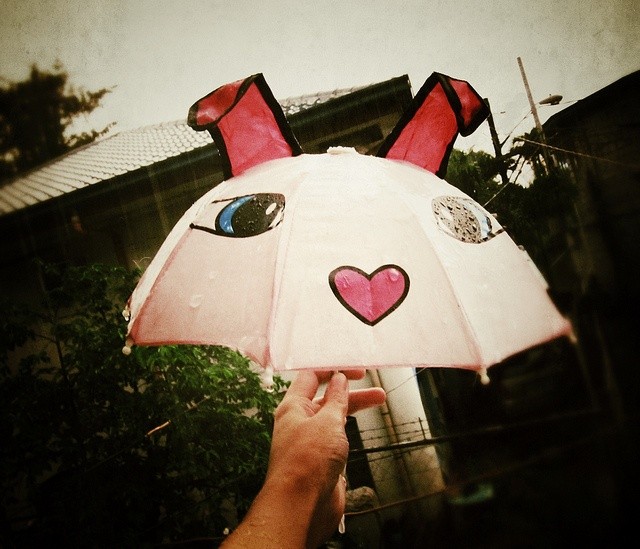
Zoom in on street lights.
[482,95,600,412]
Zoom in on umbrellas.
[120,72,567,387]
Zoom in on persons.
[218,367,389,549]
